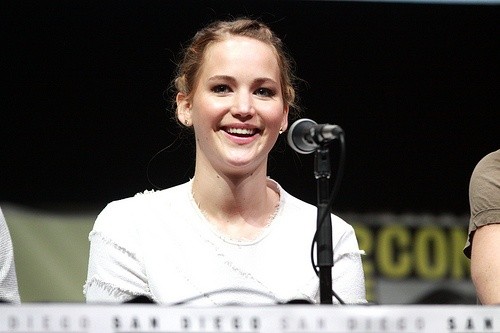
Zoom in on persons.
[81,18,370,309]
[460,148,500,306]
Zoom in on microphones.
[288,120,343,154]
